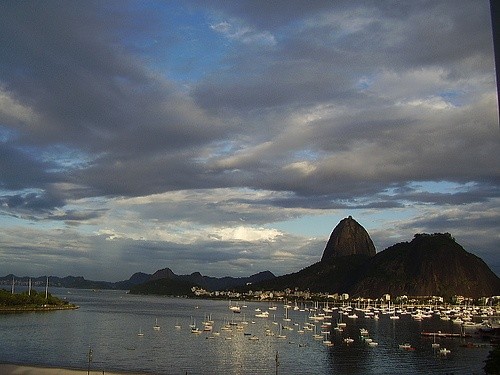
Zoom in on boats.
[136,327,145,337]
[356,300,500,331]
[297,301,356,348]
[256,300,301,344]
[190,312,261,342]
[360,328,381,349]
[228,297,247,311]
[399,342,416,352]
[431,342,453,356]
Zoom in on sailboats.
[152,316,161,331]
